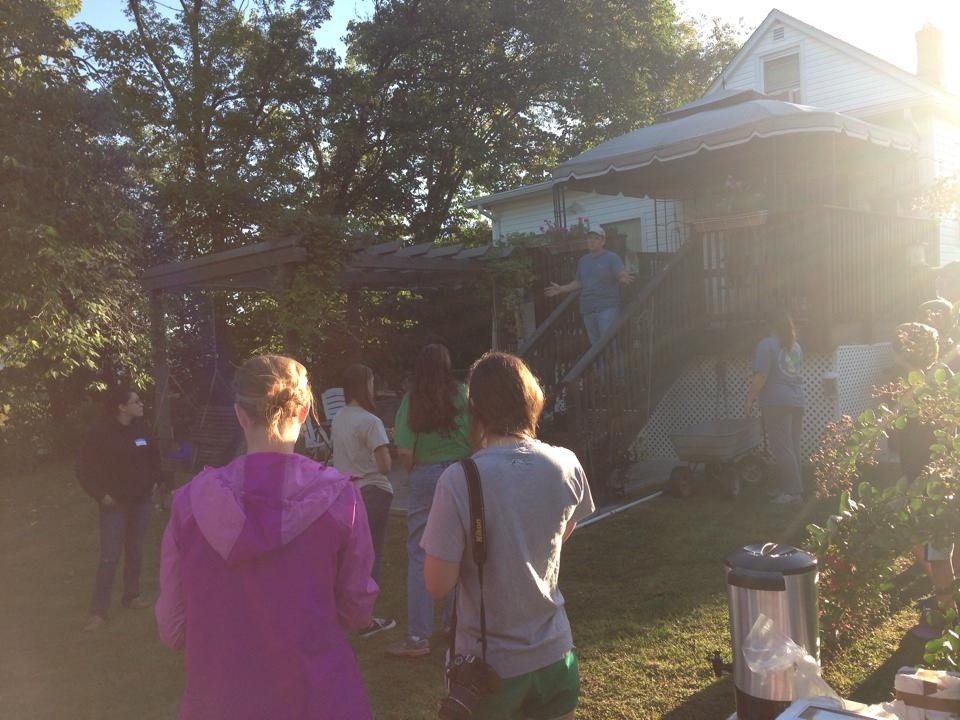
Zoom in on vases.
[685,209,769,235]
[544,231,608,257]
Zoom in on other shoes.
[360,618,397,638]
[913,626,942,638]
[387,636,430,657]
[81,612,110,632]
[917,595,939,609]
[769,494,802,505]
[128,597,151,610]
[602,382,627,397]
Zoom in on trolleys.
[664,416,769,502]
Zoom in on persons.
[890,299,960,640]
[744,304,805,505]
[544,225,631,387]
[333,344,480,656]
[81,386,161,628]
[419,351,597,720]
[156,358,380,719]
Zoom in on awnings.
[554,88,922,235]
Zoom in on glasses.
[128,399,144,405]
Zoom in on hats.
[585,225,605,237]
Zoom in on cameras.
[438,655,502,720]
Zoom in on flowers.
[539,214,593,242]
[688,168,772,218]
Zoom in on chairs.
[320,387,347,420]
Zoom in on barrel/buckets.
[711,542,823,720]
